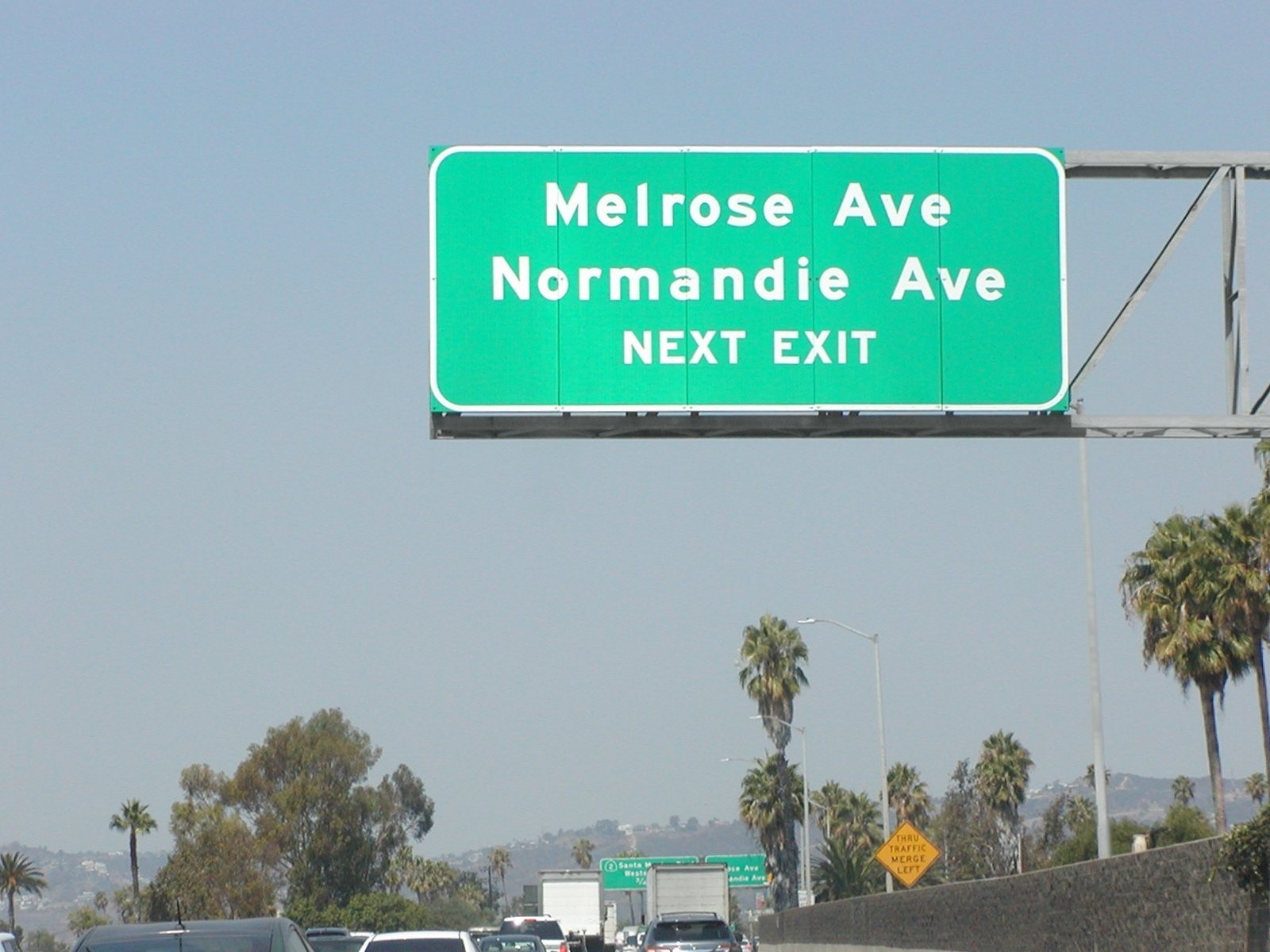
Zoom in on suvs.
[627,911,743,952]
[498,914,571,952]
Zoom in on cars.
[357,931,484,952]
[70,916,317,952]
[304,927,374,952]
[0,932,23,952]
[467,926,546,952]
[614,926,646,952]
[740,934,760,952]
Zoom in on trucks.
[537,869,617,952]
[642,862,730,927]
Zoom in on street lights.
[796,617,893,895]
[749,714,812,909]
